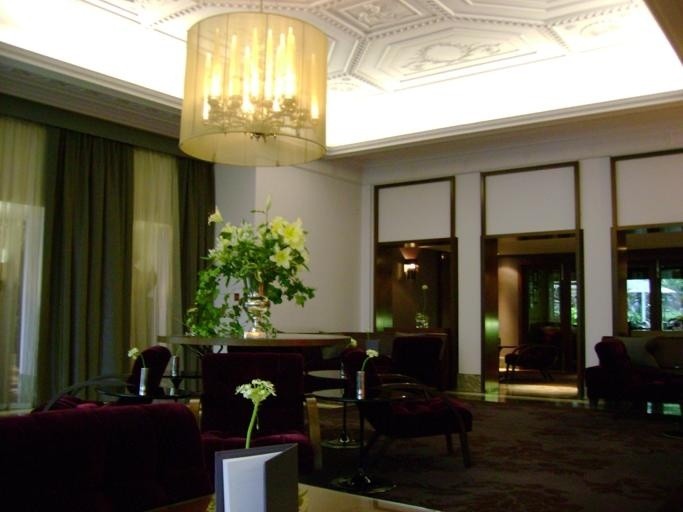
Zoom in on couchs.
[646,333,683,366]
[201,431,315,476]
[2,402,216,512]
[578,336,681,416]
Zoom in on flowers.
[129,348,146,367]
[188,194,315,337]
[362,349,378,370]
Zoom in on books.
[214,442,299,512]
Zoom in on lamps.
[402,242,421,279]
[179,11,330,166]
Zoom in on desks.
[308,367,345,382]
[165,483,441,512]
[323,395,326,397]
[315,389,366,449]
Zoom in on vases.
[139,368,148,396]
[356,371,365,400]
[241,295,271,334]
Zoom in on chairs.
[345,345,474,471]
[505,333,555,381]
[31,345,172,412]
